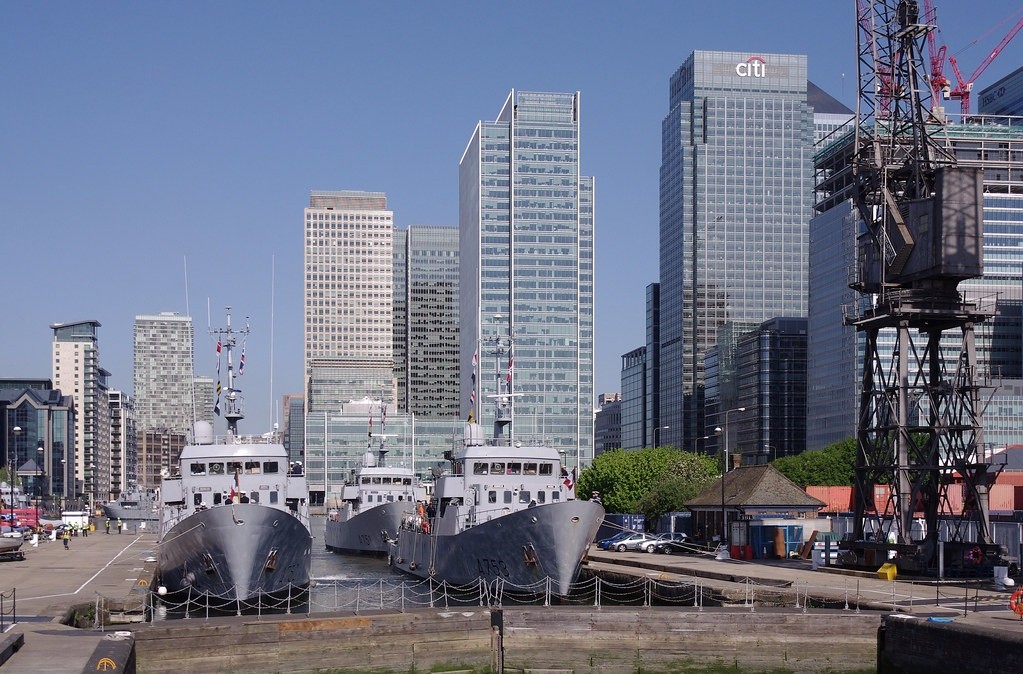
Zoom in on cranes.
[854,1,1023,198]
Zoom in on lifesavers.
[1010,591,1023,615]
[335,514,340,520]
[418,508,424,518]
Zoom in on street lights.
[765,444,777,461]
[653,425,669,450]
[60,459,67,521]
[11,426,22,532]
[715,427,724,542]
[36,446,44,526]
[726,408,746,472]
[695,436,709,456]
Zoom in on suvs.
[635,532,688,553]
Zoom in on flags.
[368,406,373,452]
[229,471,238,502]
[239,347,245,375]
[383,407,387,430]
[214,333,222,416]
[563,468,575,491]
[504,357,513,402]
[468,348,477,424]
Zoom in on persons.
[81,520,88,537]
[105,518,110,534]
[62,532,69,550]
[117,517,122,534]
[67,519,79,537]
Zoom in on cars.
[657,537,707,555]
[597,531,635,550]
[609,532,655,552]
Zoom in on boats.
[323,388,428,551]
[380,314,606,595]
[38,511,91,530]
[100,480,160,519]
[157,246,315,600]
[0,481,47,552]
